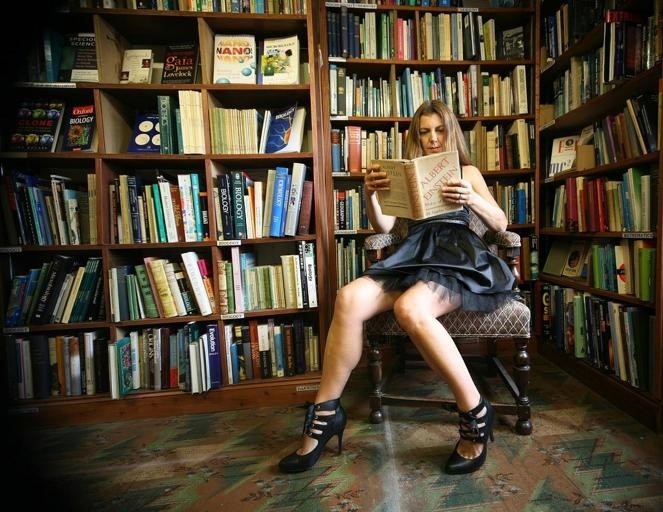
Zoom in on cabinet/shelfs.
[316,0,540,370]
[540,2,661,428]
[2,1,333,430]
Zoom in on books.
[0,2,319,399]
[541,0,663,396]
[325,2,536,296]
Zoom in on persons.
[279,98,508,474]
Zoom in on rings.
[460,194,461,199]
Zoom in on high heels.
[278,398,347,473]
[445,394,495,475]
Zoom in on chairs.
[364,232,537,437]
